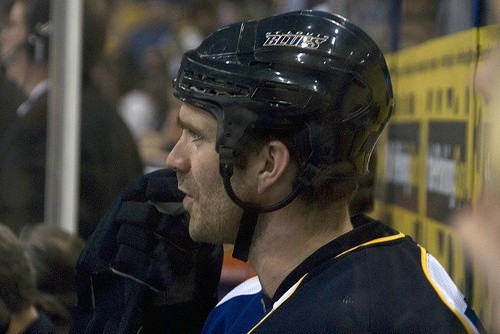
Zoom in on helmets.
[172,9,394,213]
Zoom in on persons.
[0,0,144,240]
[166,10,488,334]
[0,222,91,334]
